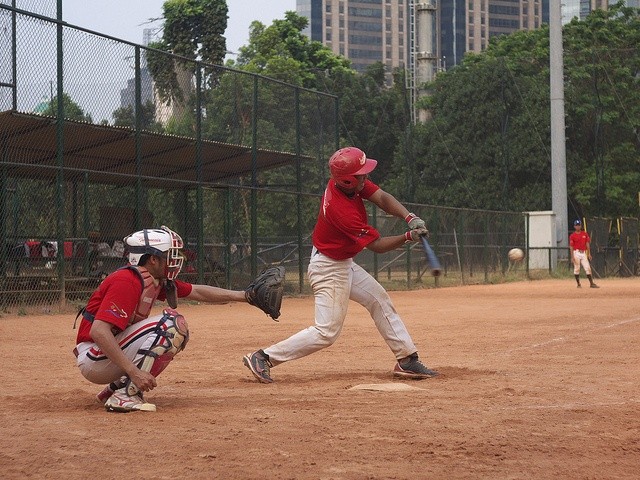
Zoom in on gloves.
[404,212,427,230]
[404,227,431,244]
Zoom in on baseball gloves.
[245,266,285,322]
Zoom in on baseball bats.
[420,235,441,277]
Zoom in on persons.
[568,219,600,287]
[72,226,247,413]
[242,146,441,384]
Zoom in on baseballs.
[508,247,524,263]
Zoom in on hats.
[574,220,581,225]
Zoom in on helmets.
[122,225,185,290]
[328,146,378,191]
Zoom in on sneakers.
[576,284,581,287]
[243,348,273,383]
[96,385,113,402]
[590,284,599,289]
[392,352,439,378]
[104,391,156,412]
